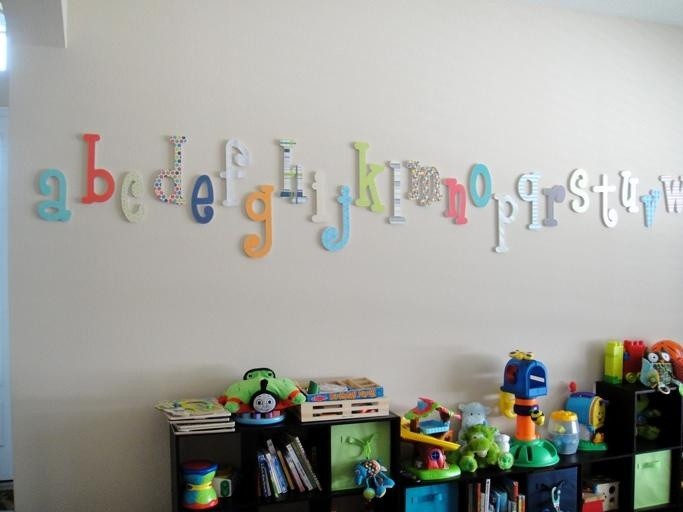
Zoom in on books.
[465,475,526,511]
[257,432,322,497]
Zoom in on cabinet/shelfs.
[594,380,683,512]
[169,409,400,511]
[400,446,632,511]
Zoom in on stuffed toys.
[450,398,510,452]
[456,422,516,472]
[218,365,306,414]
[353,456,397,501]
